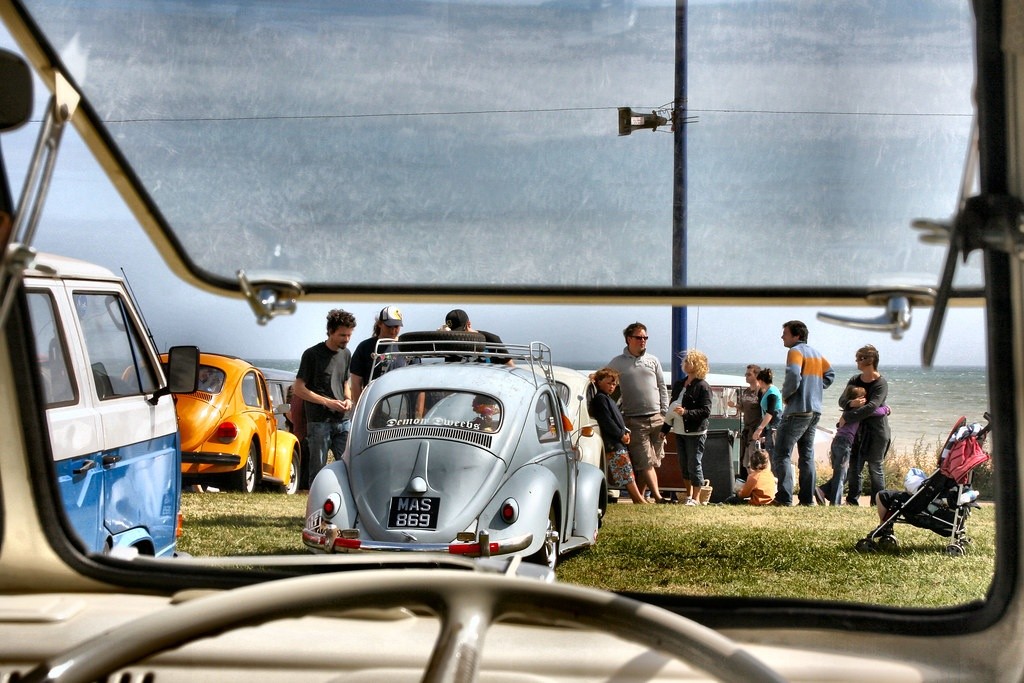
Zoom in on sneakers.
[814,486,826,506]
[684,496,699,507]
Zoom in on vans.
[9,242,202,558]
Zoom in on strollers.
[855,412,993,555]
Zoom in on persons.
[589,322,676,503]
[814,346,891,506]
[283,309,356,492]
[866,447,908,538]
[659,349,713,505]
[724,364,783,505]
[589,368,649,504]
[445,309,516,367]
[350,305,425,428]
[760,320,835,507]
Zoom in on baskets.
[699,478,713,505]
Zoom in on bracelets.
[343,398,352,402]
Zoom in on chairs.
[860,483,981,558]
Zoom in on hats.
[379,305,404,327]
[446,309,469,331]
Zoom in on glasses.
[630,336,648,340]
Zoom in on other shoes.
[868,525,894,535]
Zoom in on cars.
[121,352,302,496]
[621,369,751,492]
[243,366,297,431]
[512,363,621,503]
[301,331,608,571]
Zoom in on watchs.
[757,426,763,429]
